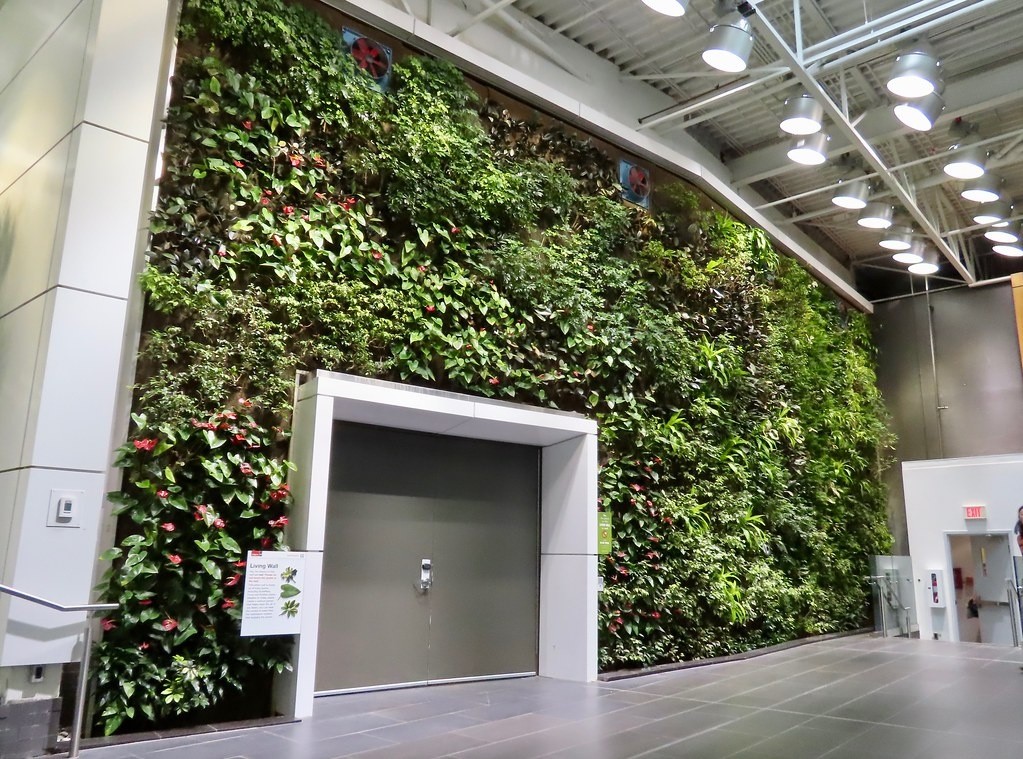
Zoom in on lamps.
[832,157,870,209]
[892,230,926,264]
[787,122,831,165]
[984,205,1021,243]
[893,67,945,132]
[943,118,987,180]
[886,30,939,99]
[991,228,1023,257]
[857,190,894,228]
[972,187,1014,228]
[641,0,690,17]
[878,212,912,250]
[960,169,1002,203]
[907,242,939,274]
[779,85,824,135]
[702,4,754,72]
